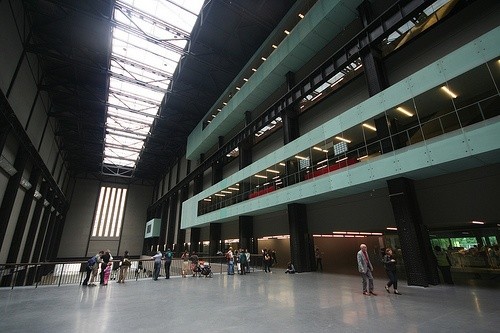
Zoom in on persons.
[216,249,222,255]
[285,262,295,274]
[116,250,130,283]
[191,251,198,277]
[486,245,498,268]
[227,247,250,275]
[153,251,162,281]
[383,249,402,295]
[263,249,277,273]
[164,248,172,279]
[434,246,453,284]
[315,247,322,270]
[83,251,112,287]
[356,244,376,296]
[181,250,189,278]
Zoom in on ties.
[364,252,369,261]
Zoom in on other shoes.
[385,285,389,291]
[363,291,368,295]
[370,291,376,295]
[394,289,399,294]
